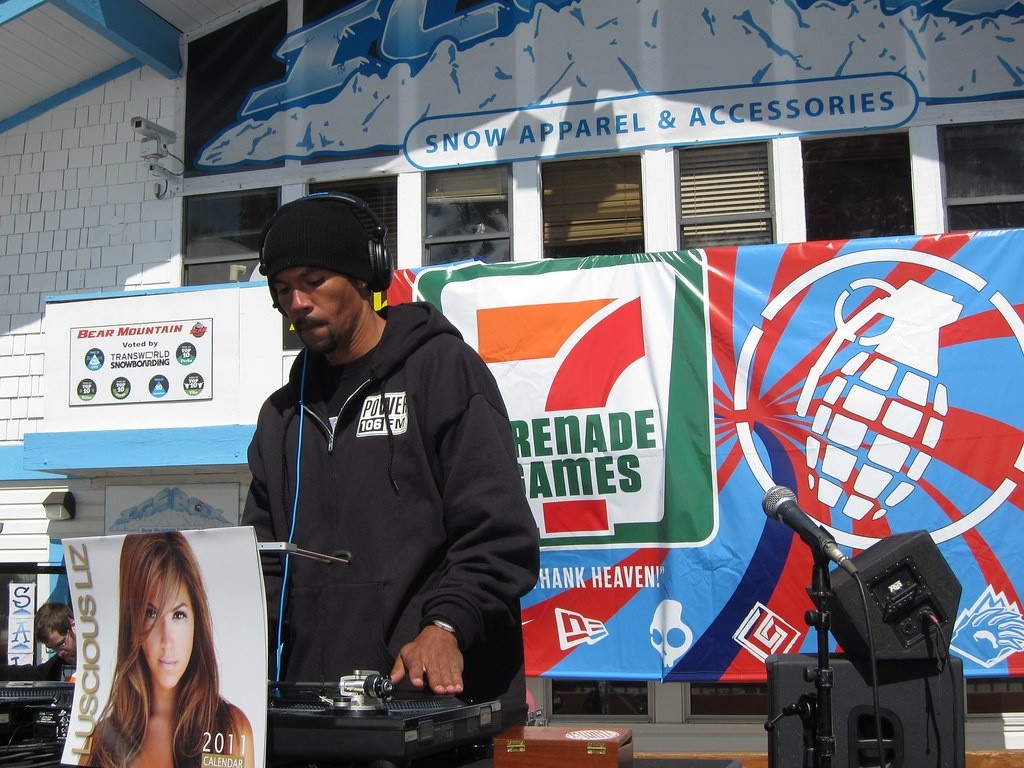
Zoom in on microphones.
[762,486,860,577]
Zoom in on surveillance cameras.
[130,116,177,143]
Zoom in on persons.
[0,603,76,682]
[78,530,255,768]
[241,198,540,725]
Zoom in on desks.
[633,750,1024,768]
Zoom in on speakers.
[804,530,962,661]
[765,653,966,768]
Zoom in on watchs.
[431,620,460,633]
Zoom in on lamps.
[42,492,75,521]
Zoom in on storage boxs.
[493,725,634,768]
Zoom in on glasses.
[46,633,68,653]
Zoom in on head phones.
[259,191,393,319]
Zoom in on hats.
[263,200,377,288]
